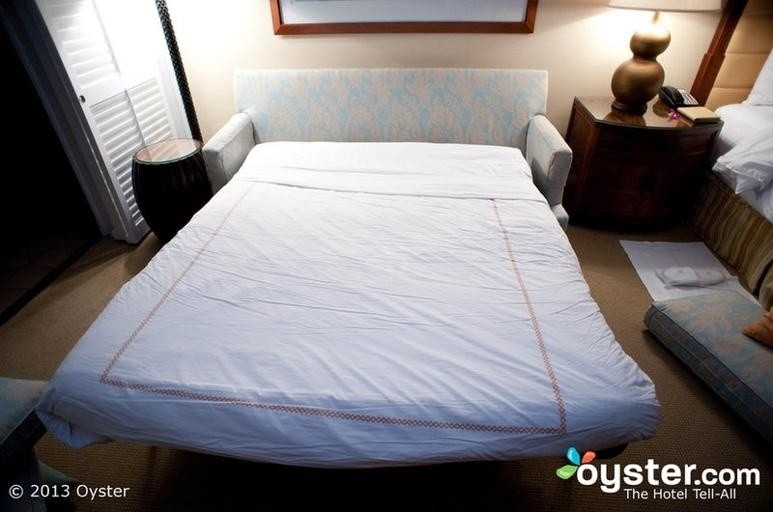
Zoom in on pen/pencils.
[679,117,693,127]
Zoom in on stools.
[132,138,215,247]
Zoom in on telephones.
[661,86,700,107]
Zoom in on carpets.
[618,240,763,308]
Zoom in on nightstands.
[564,96,724,235]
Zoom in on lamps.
[607,0,724,117]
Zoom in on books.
[676,105,721,124]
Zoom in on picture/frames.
[269,0,539,36]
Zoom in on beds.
[35,69,661,471]
